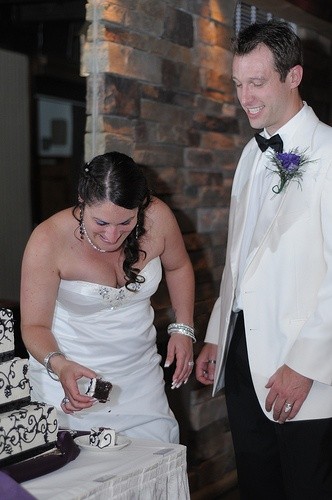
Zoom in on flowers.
[264,144,317,194]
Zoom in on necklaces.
[82,225,106,253]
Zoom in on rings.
[208,360,216,364]
[284,402,292,412]
[203,370,207,376]
[62,397,69,405]
[188,361,194,367]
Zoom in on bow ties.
[254,133,283,153]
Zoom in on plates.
[74,434,130,452]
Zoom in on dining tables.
[21,437,190,500]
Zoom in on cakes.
[0,309,58,469]
[85,378,112,403]
[88,426,115,448]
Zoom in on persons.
[19,151,198,446]
[195,23,332,500]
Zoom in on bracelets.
[167,324,196,344]
[44,351,68,382]
